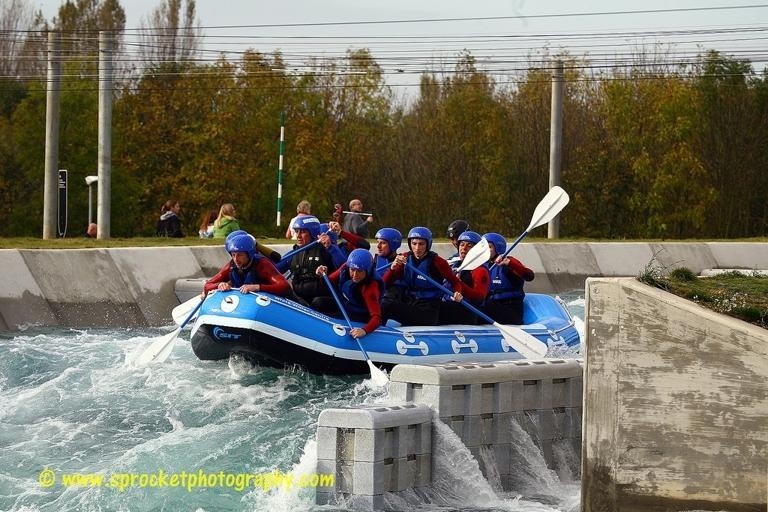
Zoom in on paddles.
[442,239,491,283]
[141,289,207,363]
[319,268,391,388]
[489,186,570,270]
[171,238,324,325]
[402,260,548,360]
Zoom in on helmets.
[320,222,338,245]
[482,233,507,255]
[458,231,480,244]
[447,220,470,237]
[374,228,403,252]
[227,235,256,262]
[292,215,319,243]
[407,226,433,253]
[225,230,249,256]
[346,248,374,278]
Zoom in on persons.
[204,235,291,295]
[380,226,463,327]
[342,199,374,237]
[199,208,219,239]
[213,204,241,239]
[444,219,473,260]
[312,248,381,339]
[285,201,319,248]
[273,216,347,309]
[481,232,535,325]
[370,228,403,281]
[225,229,280,266]
[320,220,371,268]
[440,231,491,325]
[154,199,186,238]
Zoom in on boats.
[190,285,583,377]
[190,292,579,373]
